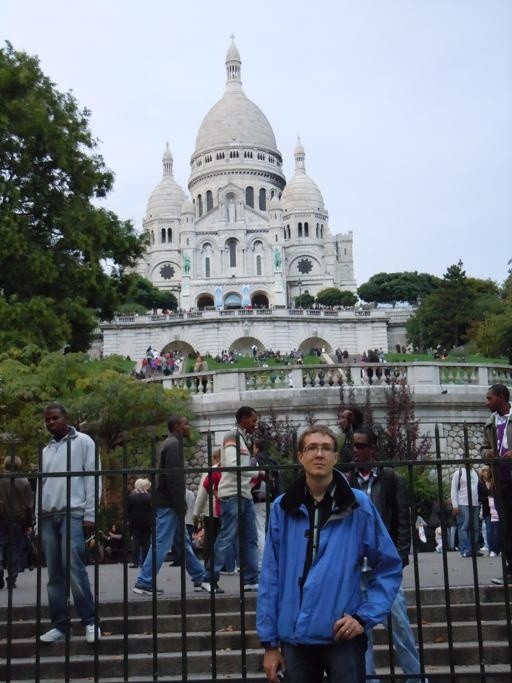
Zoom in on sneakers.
[462,552,484,557]
[85,623,102,644]
[193,579,228,594]
[38,626,72,642]
[244,582,263,592]
[131,582,166,596]
[491,574,512,586]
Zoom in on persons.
[161,303,354,319]
[480,385,512,585]
[182,253,191,275]
[227,199,236,224]
[272,247,282,271]
[34,404,103,644]
[343,426,428,683]
[256,425,403,683]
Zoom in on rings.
[346,630,350,634]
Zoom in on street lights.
[176,281,183,310]
[297,278,302,309]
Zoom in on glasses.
[353,442,374,450]
[303,445,335,453]
